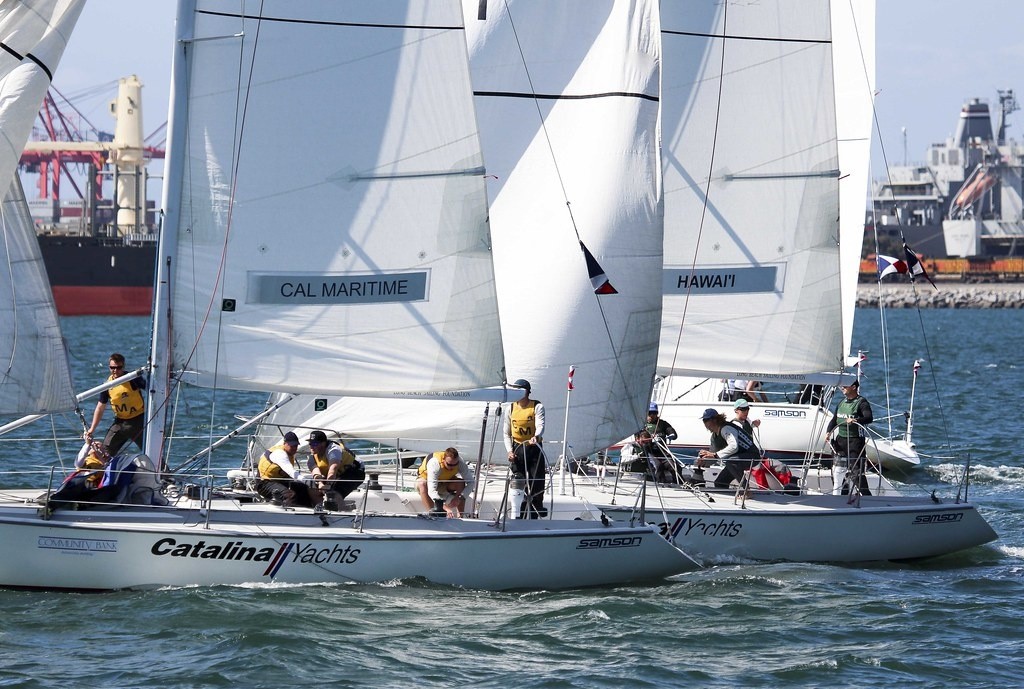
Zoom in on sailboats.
[0,0,707,589]
[229,0,1001,565]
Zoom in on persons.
[254,432,316,509]
[719,379,769,402]
[698,409,761,489]
[85,353,146,459]
[730,398,761,442]
[307,431,366,510]
[414,447,475,518]
[827,382,872,496]
[637,402,688,484]
[793,383,824,407]
[619,430,653,476]
[399,448,416,468]
[502,379,545,520]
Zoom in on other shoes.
[744,487,753,498]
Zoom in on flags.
[580,240,619,295]
[905,244,938,290]
[879,254,908,278]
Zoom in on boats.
[14,62,168,316]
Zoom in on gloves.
[667,433,675,440]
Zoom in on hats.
[700,408,718,419]
[508,379,531,393]
[284,432,300,446]
[734,399,753,410]
[852,380,858,385]
[648,403,657,411]
[306,431,327,442]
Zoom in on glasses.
[309,441,322,446]
[642,441,648,445]
[445,460,459,466]
[649,411,657,415]
[737,406,749,411]
[110,365,123,370]
